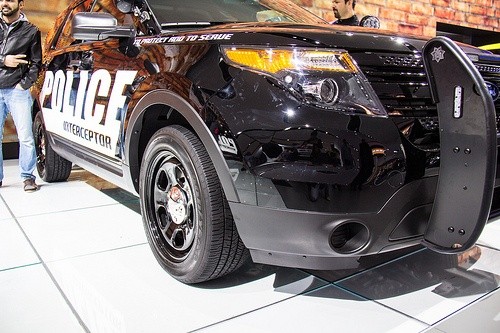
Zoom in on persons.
[0,0,42,191]
[329,0,360,26]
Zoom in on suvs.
[30,0,499,285]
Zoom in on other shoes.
[0,179,4,187]
[24,178,38,191]
